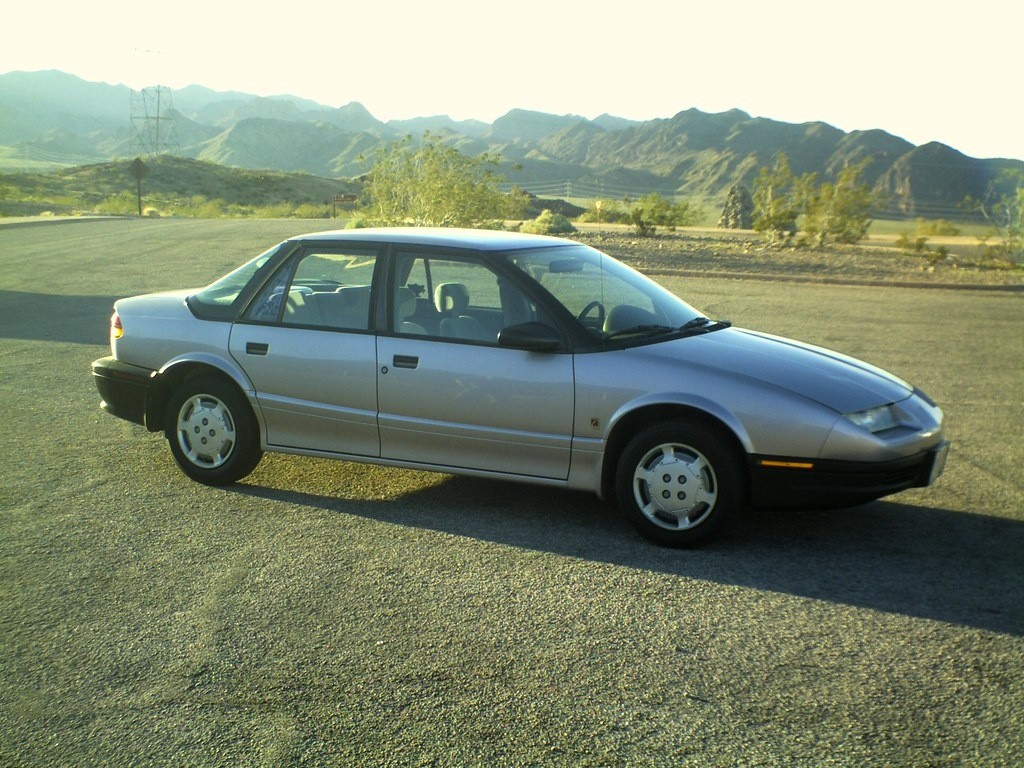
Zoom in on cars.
[92,227,951,549]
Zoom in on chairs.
[398,288,426,334]
[435,282,486,341]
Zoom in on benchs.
[270,286,372,327]
